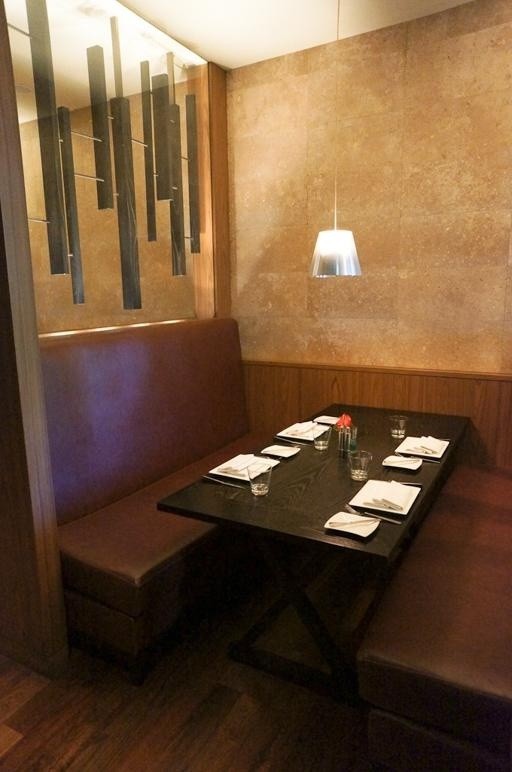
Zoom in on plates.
[276,422,330,441]
[313,414,340,426]
[260,445,301,459]
[348,479,422,516]
[394,436,450,459]
[323,511,381,538]
[381,455,423,470]
[208,454,281,482]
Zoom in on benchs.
[352,454,509,769]
[37,317,277,689]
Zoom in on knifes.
[201,474,245,489]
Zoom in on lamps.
[308,0,363,281]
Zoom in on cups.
[312,426,332,451]
[247,463,272,496]
[348,450,371,481]
[389,415,408,439]
[339,425,357,451]
